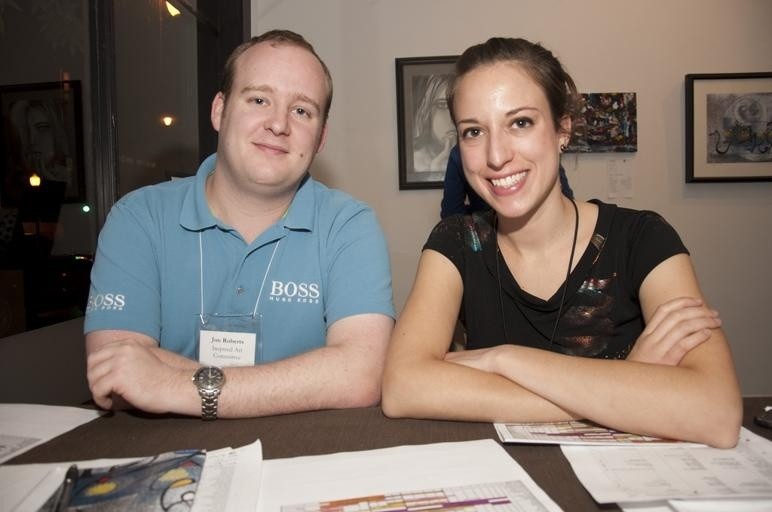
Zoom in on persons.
[84,31,395,419]
[381,37,744,450]
[10,87,84,195]
[439,144,574,220]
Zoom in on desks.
[0,396,771,512]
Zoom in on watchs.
[192,366,225,419]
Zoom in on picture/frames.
[399,57,462,191]
[687,73,772,183]
[0,82,86,205]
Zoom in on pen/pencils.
[52,464,78,512]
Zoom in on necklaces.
[496,199,579,348]
